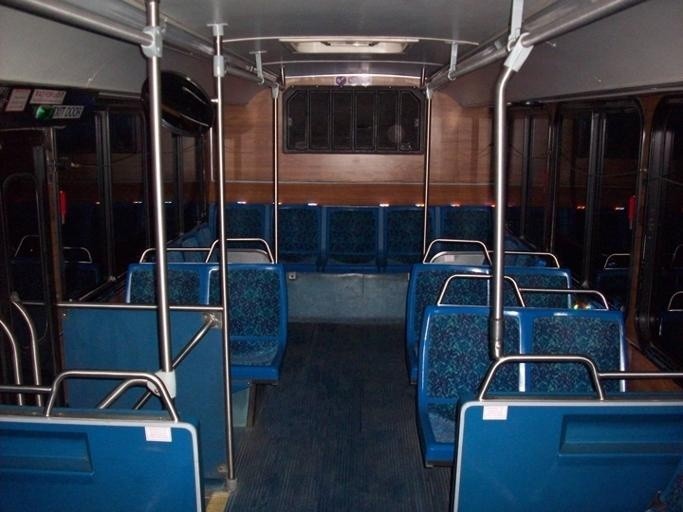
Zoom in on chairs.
[126,202,627,470]
